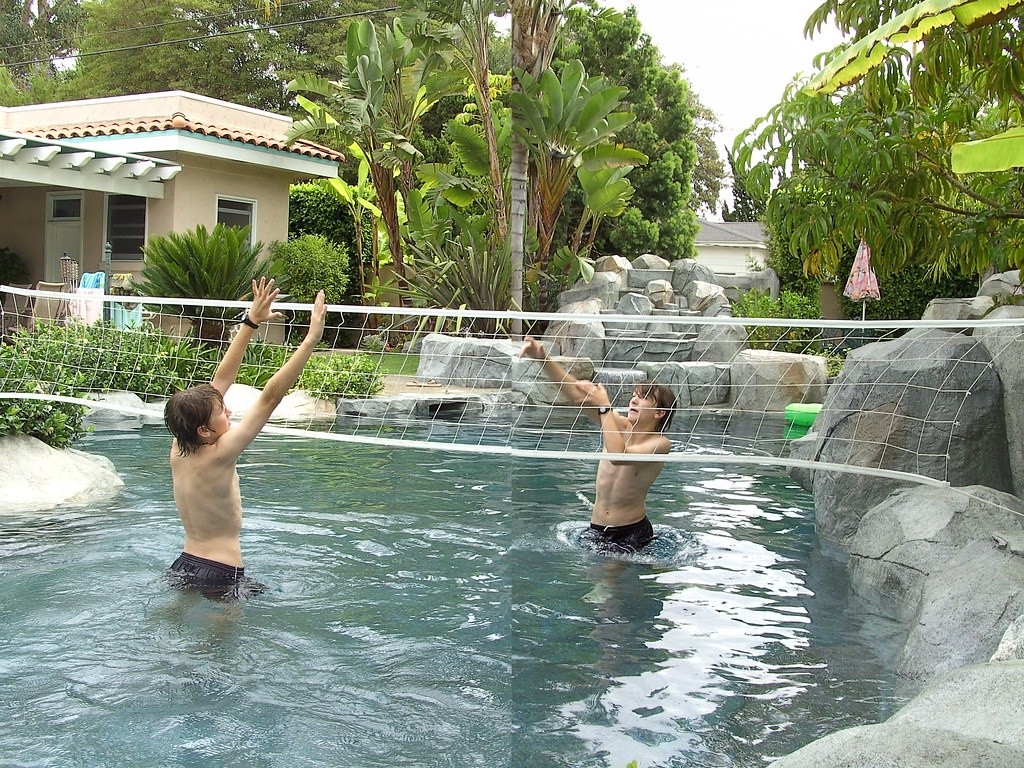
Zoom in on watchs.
[241,313,259,329]
[598,408,613,415]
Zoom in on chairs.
[0,283,32,335]
[30,281,68,325]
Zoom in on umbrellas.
[843,227,881,346]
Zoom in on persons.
[517,335,678,587]
[164,275,328,631]
